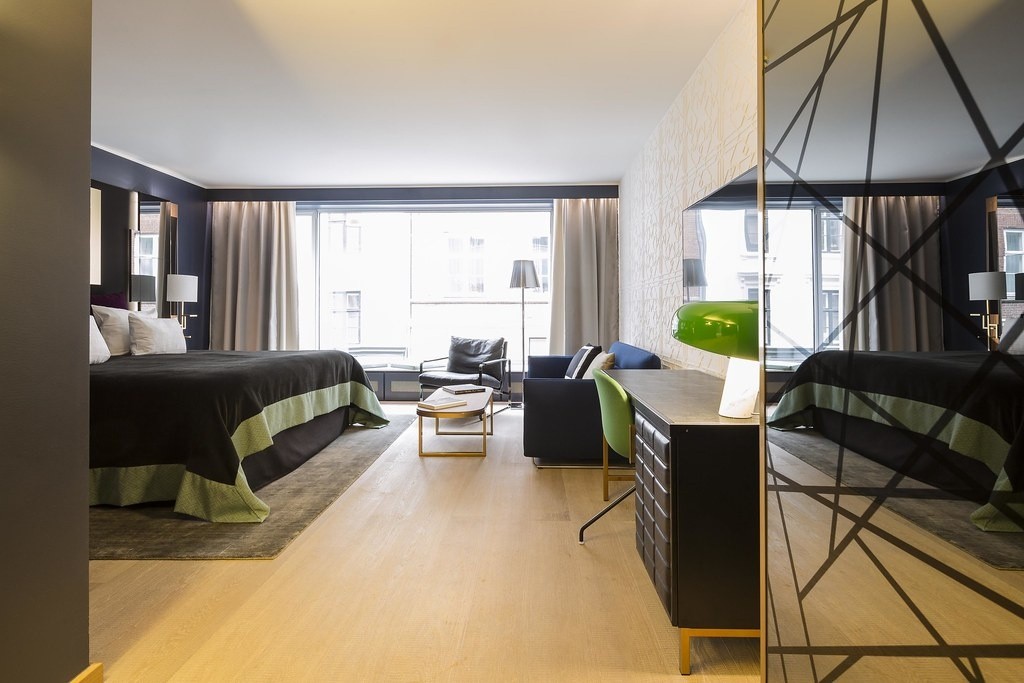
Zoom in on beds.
[767,349,1023,534]
[89,349,390,523]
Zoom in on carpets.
[768,398,1022,572]
[88,413,420,560]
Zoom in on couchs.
[523,340,663,470]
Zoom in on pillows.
[565,343,602,379]
[128,313,188,356]
[583,350,615,379]
[90,314,112,366]
[91,303,160,357]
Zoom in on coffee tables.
[417,383,496,458]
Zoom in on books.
[442,384,485,394]
[418,397,467,410]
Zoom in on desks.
[766,367,800,402]
[603,368,763,676]
[360,362,529,403]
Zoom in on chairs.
[578,366,636,543]
[418,336,512,422]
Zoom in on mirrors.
[762,0,1024,682]
[988,198,1024,355]
[130,190,179,319]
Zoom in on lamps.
[1015,273,1024,301]
[671,301,762,421]
[510,257,541,409]
[165,274,199,340]
[683,258,709,301]
[131,275,156,311]
[967,272,1008,351]
